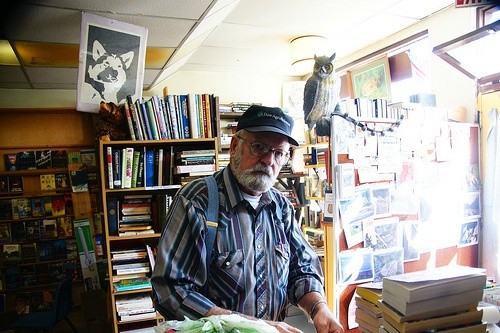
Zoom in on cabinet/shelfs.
[0,145,106,294]
[219,112,309,190]
[98,137,218,333]
[303,142,330,201]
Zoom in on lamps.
[288,34,329,68]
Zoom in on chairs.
[11,277,81,333]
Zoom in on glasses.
[236,135,290,166]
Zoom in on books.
[96,88,317,320]
[354,259,490,333]
[0,150,109,333]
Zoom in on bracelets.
[309,301,327,319]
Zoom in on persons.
[151,103,345,333]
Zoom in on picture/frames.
[345,54,392,101]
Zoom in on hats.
[236,105,299,146]
